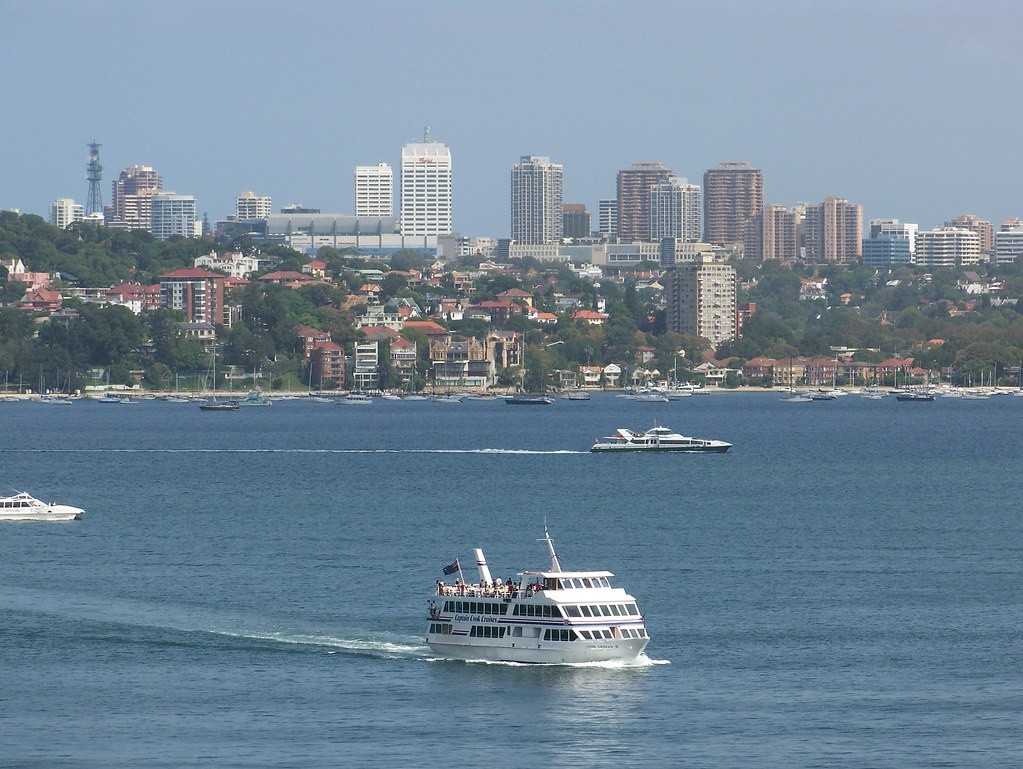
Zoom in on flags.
[443,560,458,575]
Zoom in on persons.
[432,600,437,614]
[480,579,483,587]
[427,600,433,616]
[485,581,488,588]
[440,580,443,586]
[436,580,439,590]
[508,582,542,591]
[496,577,503,585]
[506,578,512,585]
[493,579,497,588]
[456,578,459,584]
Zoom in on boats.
[423,524,649,665]
[590,417,733,452]
[0,488,85,520]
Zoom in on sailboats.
[779,360,1023,402]
[615,354,711,401]
[0,332,591,411]
